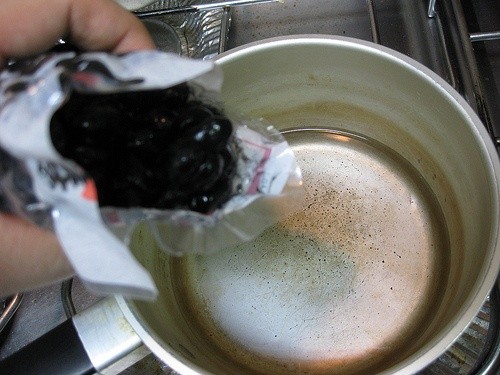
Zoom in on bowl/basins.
[112,33,499,374]
